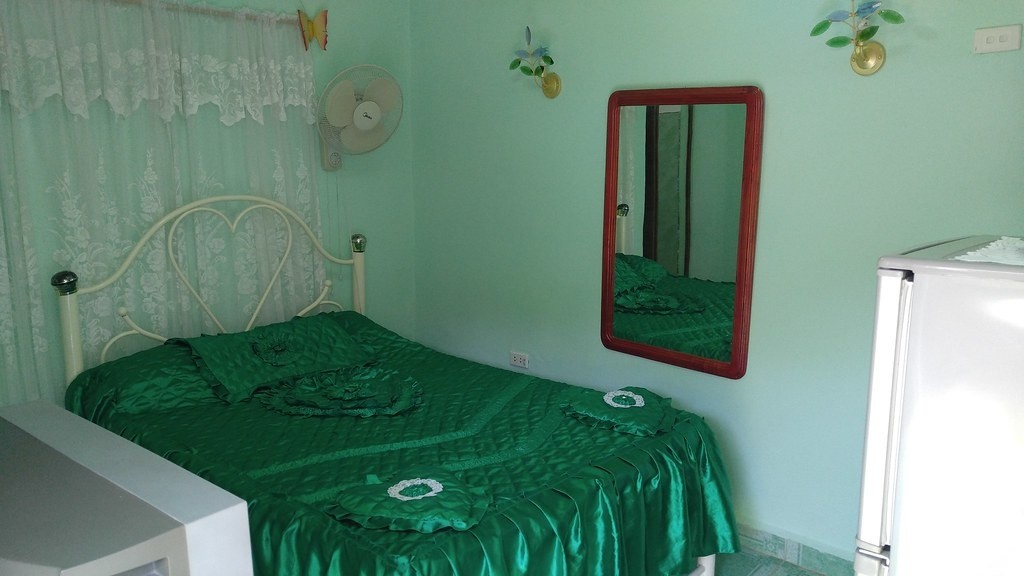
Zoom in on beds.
[614,204,735,364]
[50,195,739,576]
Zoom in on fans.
[315,63,403,171]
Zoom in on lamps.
[510,25,561,98]
[810,0,905,77]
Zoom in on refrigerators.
[853,233,1024,576]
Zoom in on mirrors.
[601,86,764,379]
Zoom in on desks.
[0,397,254,576]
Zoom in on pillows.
[561,385,674,437]
[613,255,655,298]
[323,463,491,534]
[173,312,381,404]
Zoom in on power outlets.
[510,351,527,368]
